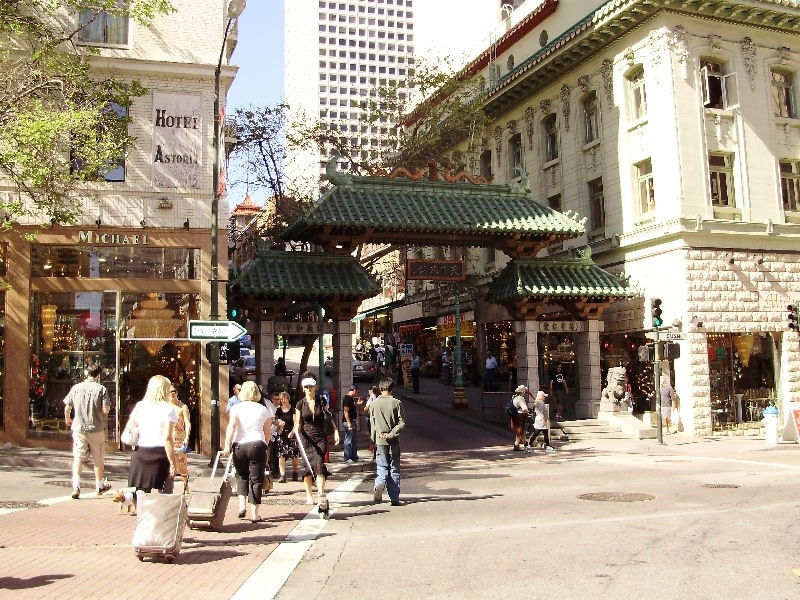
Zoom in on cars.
[230,355,279,384]
[231,335,251,366]
[324,351,377,383]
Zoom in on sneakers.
[72,488,81,499]
[97,483,111,495]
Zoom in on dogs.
[113,489,137,513]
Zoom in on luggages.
[186,451,233,529]
[134,474,189,562]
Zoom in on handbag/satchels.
[322,412,334,436]
[263,465,273,490]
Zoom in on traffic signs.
[188,320,248,343]
[205,341,240,360]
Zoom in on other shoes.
[346,459,353,464]
[374,483,385,503]
[317,506,329,519]
[556,415,563,422]
[514,445,520,451]
[526,446,532,452]
[251,516,261,523]
[278,476,287,483]
[352,457,359,462]
[392,501,403,506]
[546,446,555,453]
[239,510,246,518]
[292,472,299,481]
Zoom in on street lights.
[206,0,247,467]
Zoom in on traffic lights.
[663,343,680,360]
[225,283,244,319]
[651,298,663,327]
[637,345,653,363]
[787,303,800,332]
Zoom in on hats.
[537,391,548,398]
[301,378,317,387]
[515,385,527,393]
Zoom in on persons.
[525,391,557,453]
[62,340,425,523]
[223,381,272,523]
[659,375,676,435]
[483,350,499,392]
[442,347,452,385]
[550,361,569,421]
[366,377,406,506]
[511,385,532,453]
[127,374,177,515]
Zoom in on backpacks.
[505,396,521,417]
